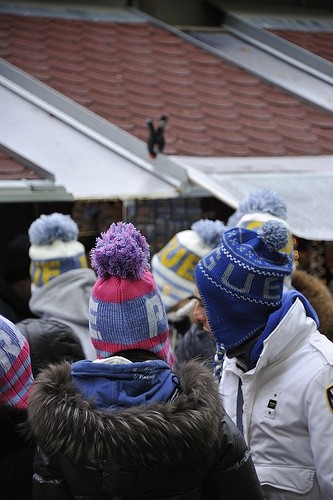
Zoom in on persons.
[0,213,99,500]
[150,190,333,500]
[33,223,268,500]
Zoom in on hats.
[29,213,87,294]
[89,221,174,370]
[151,219,225,309]
[0,315,35,409]
[195,220,293,385]
[228,189,295,275]
